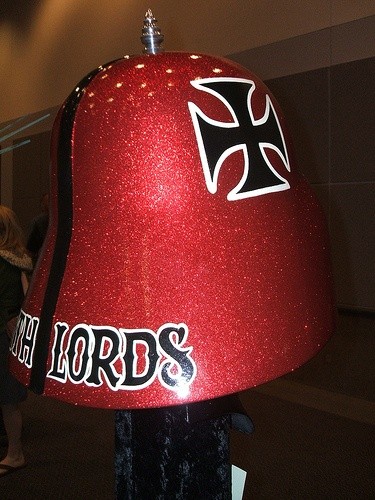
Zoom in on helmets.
[7,7,337,410]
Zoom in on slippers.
[0,458,30,475]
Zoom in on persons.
[26,195,50,265]
[0,205,34,478]
